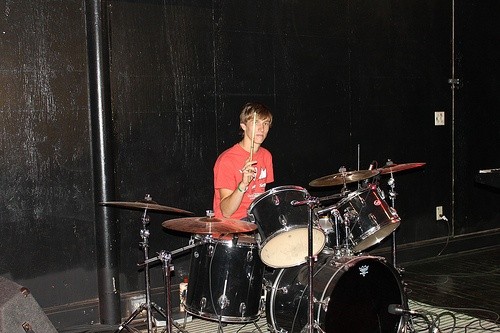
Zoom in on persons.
[213,101,275,241]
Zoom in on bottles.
[180,277,188,312]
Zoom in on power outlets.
[437,206,443,221]
[435,111,444,125]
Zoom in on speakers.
[0,274,59,333]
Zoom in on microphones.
[387,303,434,316]
[361,160,375,188]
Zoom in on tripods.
[114,209,189,333]
[290,179,348,333]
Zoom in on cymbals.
[377,159,427,174]
[100,193,194,215]
[308,166,379,187]
[161,209,258,234]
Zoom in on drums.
[265,252,408,333]
[328,186,401,253]
[185,234,265,323]
[246,184,326,269]
